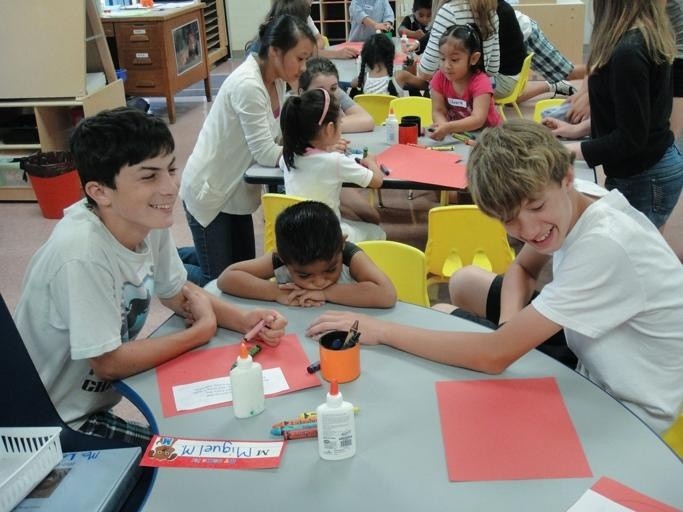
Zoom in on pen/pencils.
[330,320,361,350]
[270,406,360,440]
[428,146,455,150]
[409,143,427,148]
[380,163,389,175]
[229,345,261,369]
[428,128,435,132]
[307,360,320,373]
[243,311,277,343]
[363,146,368,157]
[451,131,478,145]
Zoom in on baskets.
[1,426,65,512]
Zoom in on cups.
[318,330,362,385]
[383,31,392,41]
[401,115,421,137]
[399,122,419,145]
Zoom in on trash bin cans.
[20,151,82,218]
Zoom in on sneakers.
[547,78,578,98]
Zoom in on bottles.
[316,379,355,461]
[400,30,409,50]
[384,107,399,145]
[228,342,266,420]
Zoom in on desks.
[100,3,212,125]
[112,277,683,512]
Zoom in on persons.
[12,105,289,446]
[216,201,396,309]
[306,118,682,433]
[175,0,683,287]
